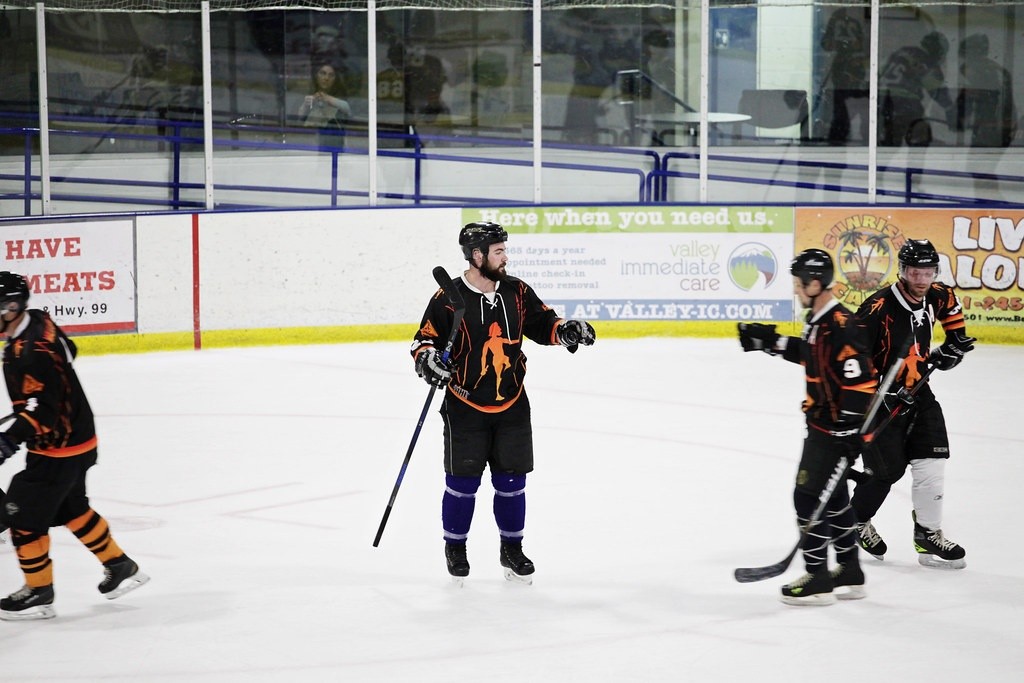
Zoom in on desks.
[634,113,751,146]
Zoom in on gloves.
[928,330,977,371]
[0,432,20,464]
[557,320,596,348]
[944,108,966,133]
[738,322,780,356]
[878,373,916,417]
[417,351,459,389]
[828,418,863,467]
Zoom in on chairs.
[906,87,1018,147]
[727,89,812,146]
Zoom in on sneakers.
[912,511,966,569]
[499,537,535,584]
[0,581,55,620]
[855,521,887,561]
[445,537,470,586]
[97,555,150,599]
[828,544,865,600]
[781,566,837,606]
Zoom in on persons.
[555,0,688,149]
[848,238,968,570]
[737,247,885,607]
[0,270,149,618]
[794,5,1017,204]
[375,42,407,116]
[410,222,595,589]
[295,63,349,152]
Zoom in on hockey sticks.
[372,267,464,546]
[736,331,916,584]
[839,362,939,454]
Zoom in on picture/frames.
[865,0,920,21]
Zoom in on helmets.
[315,26,337,51]
[896,239,939,274]
[459,222,508,260]
[959,35,988,61]
[180,34,199,63]
[790,249,834,289]
[923,29,949,65]
[0,271,29,310]
